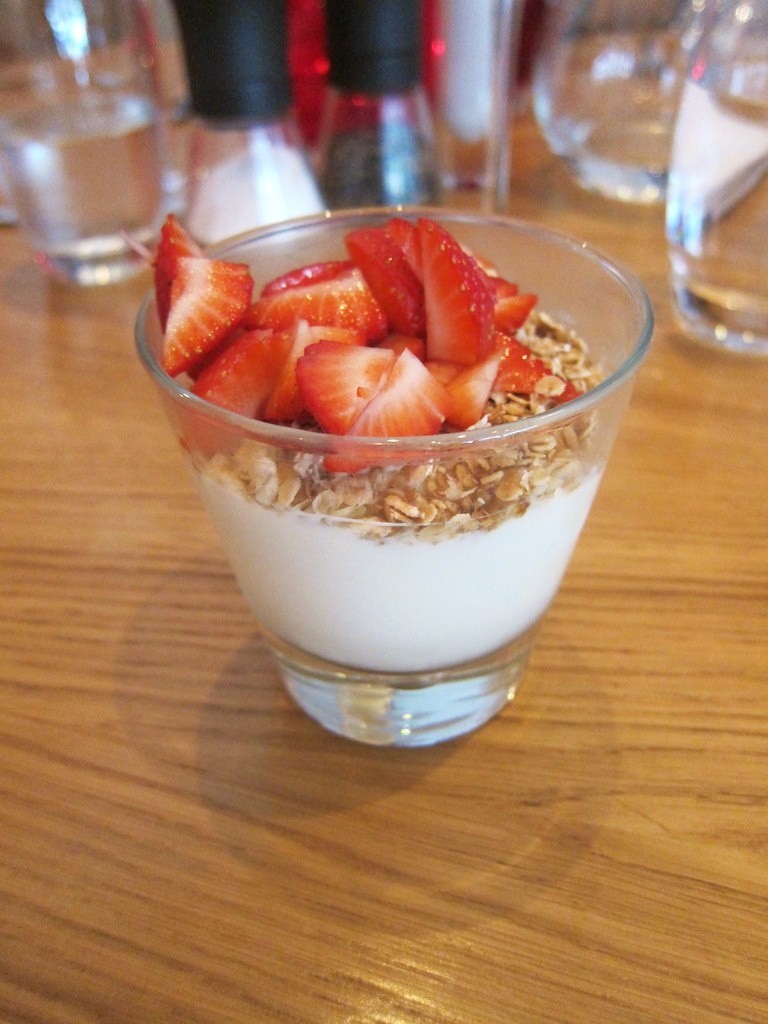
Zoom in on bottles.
[172,0,329,249]
[310,0,446,212]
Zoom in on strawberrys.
[154,211,585,470]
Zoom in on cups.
[0,0,168,287]
[428,1,527,213]
[664,0,768,357]
[134,206,655,747]
[534,0,675,202]
